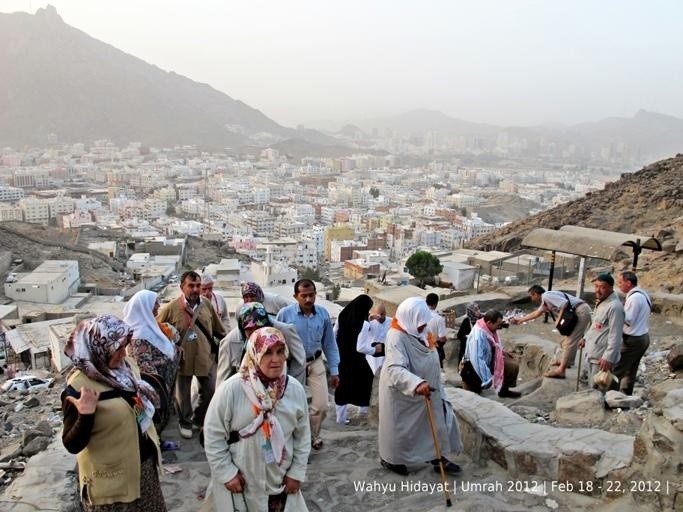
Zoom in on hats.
[598,272,614,286]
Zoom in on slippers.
[551,360,574,366]
[160,440,180,450]
[543,371,566,379]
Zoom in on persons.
[199,272,231,334]
[60,315,167,511]
[276,278,341,449]
[425,293,447,369]
[203,326,312,512]
[578,274,625,394]
[215,301,306,395]
[377,297,464,473]
[123,290,185,450]
[605,272,652,411]
[235,282,288,323]
[458,303,521,398]
[512,285,592,378]
[332,294,395,424]
[159,272,227,438]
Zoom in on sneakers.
[424,456,461,473]
[380,459,409,476]
[311,439,329,450]
[498,391,521,399]
[179,423,192,439]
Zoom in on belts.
[306,350,322,363]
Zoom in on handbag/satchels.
[140,371,171,435]
[555,307,579,336]
